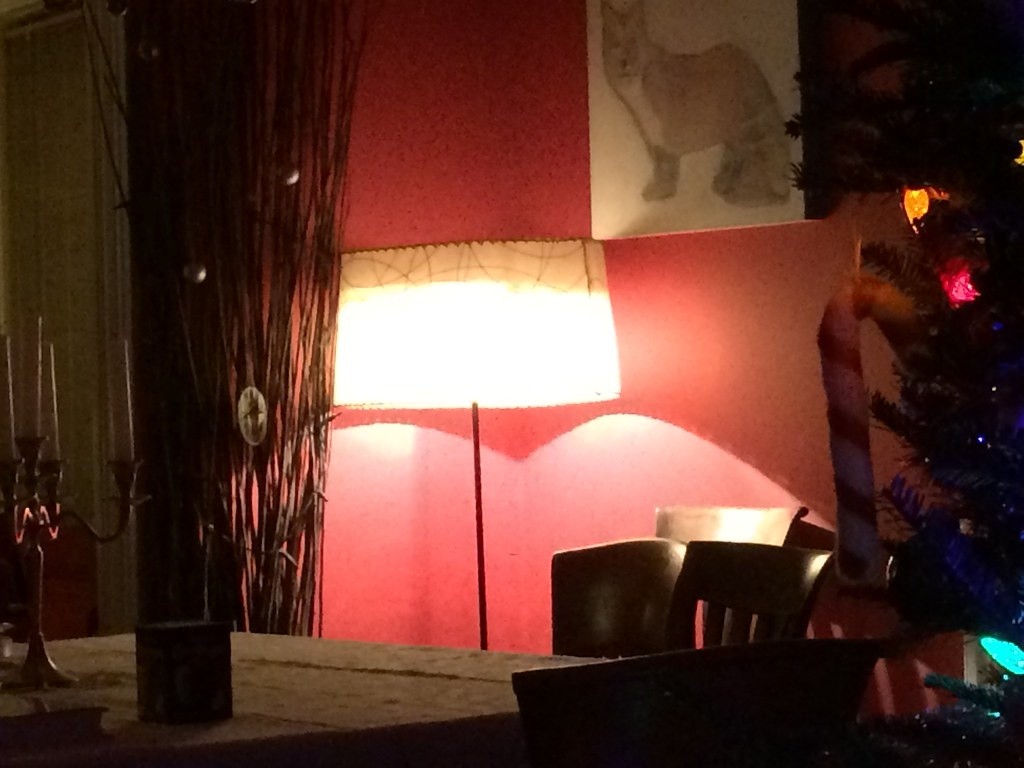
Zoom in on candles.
[108,333,135,462]
[22,311,44,437]
[42,341,59,460]
[0,334,17,463]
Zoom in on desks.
[0,632,606,768]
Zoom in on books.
[1,680,113,748]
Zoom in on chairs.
[509,505,890,768]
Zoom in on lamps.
[331,240,623,650]
